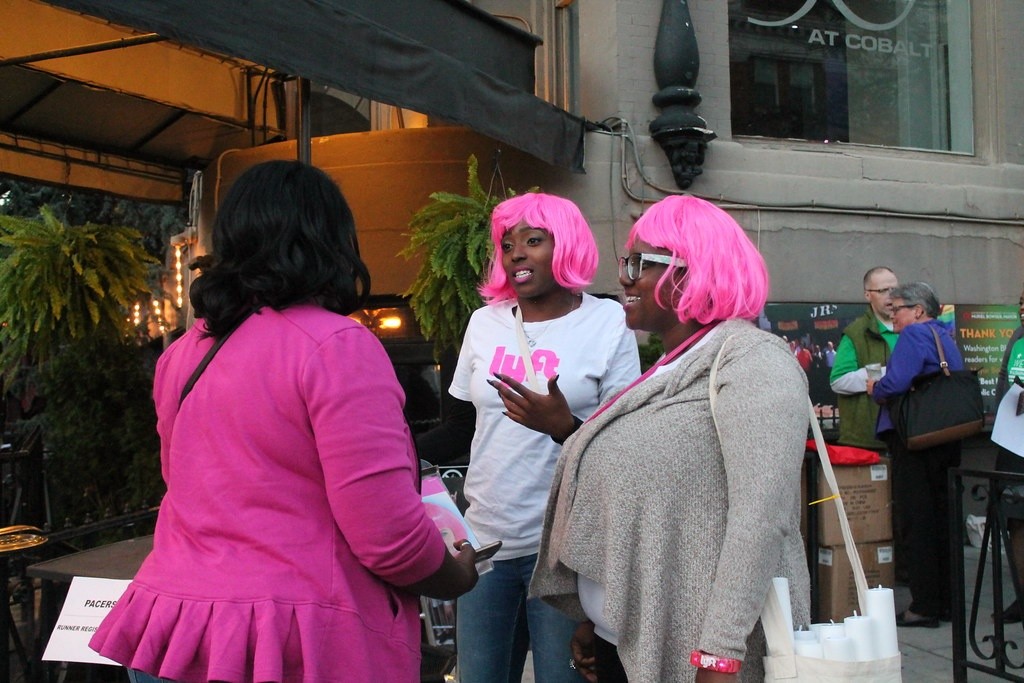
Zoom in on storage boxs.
[813,544,895,623]
[805,459,894,546]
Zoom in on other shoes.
[992,598,1022,625]
[896,608,940,627]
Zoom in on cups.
[865,363,883,382]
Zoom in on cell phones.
[472,541,502,563]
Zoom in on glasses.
[891,303,926,314]
[618,252,688,286]
[865,286,895,293]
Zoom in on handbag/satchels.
[967,514,1004,552]
[764,646,902,682]
[899,323,987,450]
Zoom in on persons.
[830,267,904,456]
[89,159,480,683]
[867,282,965,629]
[782,332,838,406]
[991,292,1024,628]
[530,195,810,683]
[449,194,642,683]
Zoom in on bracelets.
[690,650,742,673]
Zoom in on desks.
[28,534,158,683]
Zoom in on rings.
[461,542,471,546]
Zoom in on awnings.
[0,0,588,178]
